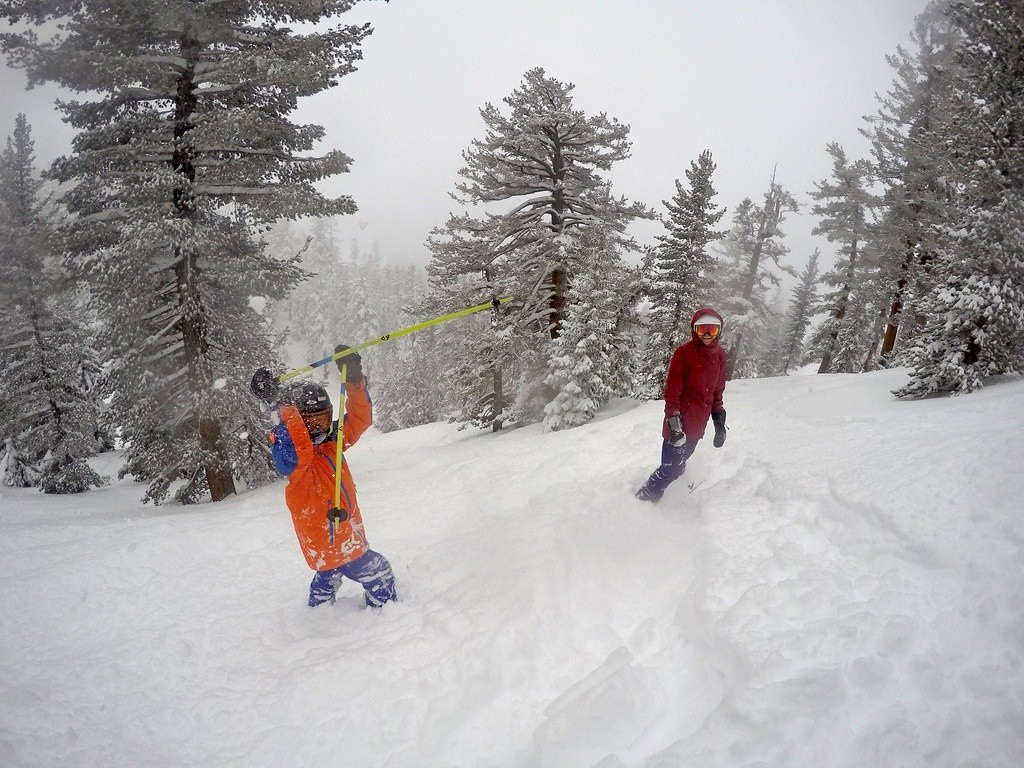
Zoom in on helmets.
[280,381,333,416]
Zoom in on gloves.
[667,414,687,447]
[249,366,292,411]
[335,344,363,382]
[712,410,726,448]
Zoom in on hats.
[693,314,721,335]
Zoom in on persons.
[249,343,398,608]
[636,308,728,501]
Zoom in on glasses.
[696,324,720,336]
[299,408,332,433]
